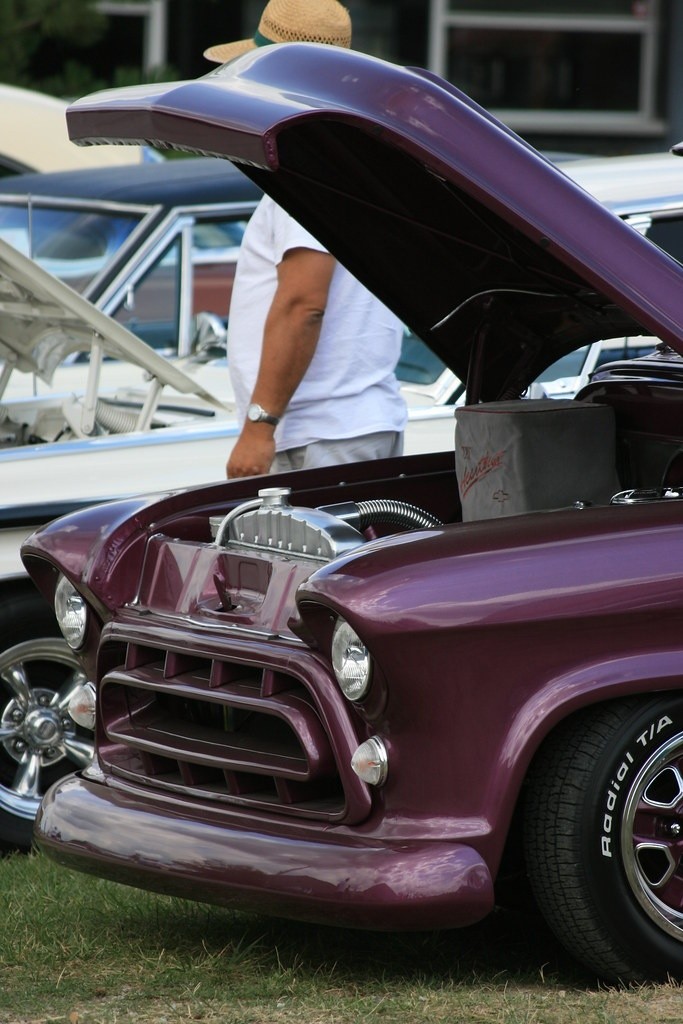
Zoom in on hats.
[203,0,352,65]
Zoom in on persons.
[204,0,410,479]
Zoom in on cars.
[0,40,683,998]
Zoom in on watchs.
[247,403,280,425]
